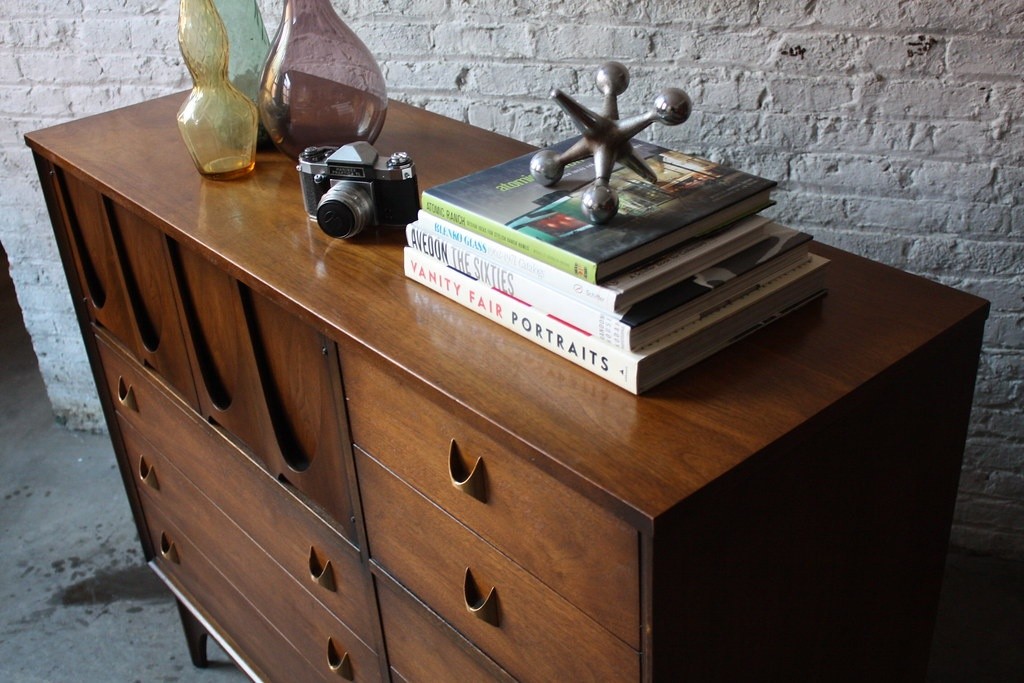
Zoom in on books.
[402,133,837,397]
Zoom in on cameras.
[295,140,420,240]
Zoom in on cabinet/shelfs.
[25,69,992,683]
